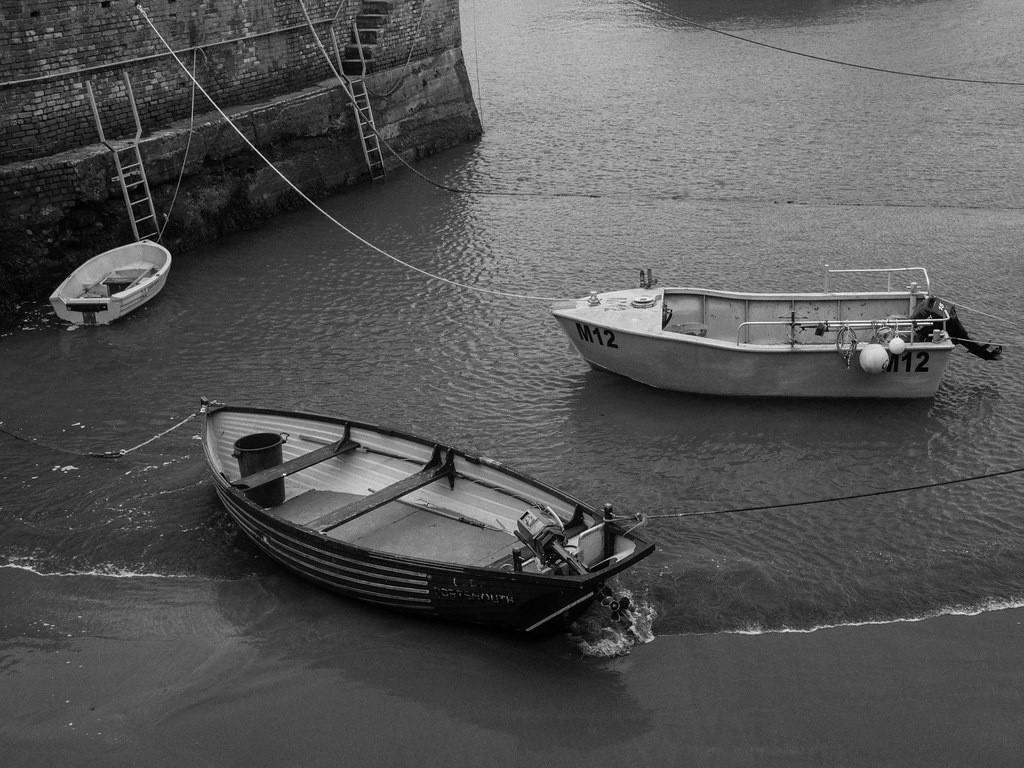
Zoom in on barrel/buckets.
[231,432,289,509]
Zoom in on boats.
[550,265,956,401]
[48,237,174,331]
[195,393,658,643]
